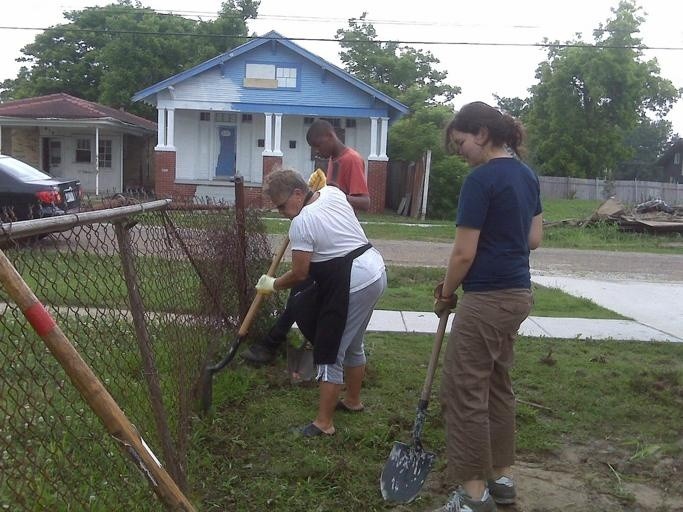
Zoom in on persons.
[302,119,374,213]
[253,166,390,439]
[431,101,543,512]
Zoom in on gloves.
[308,168,327,193]
[433,281,457,318]
[255,273,278,295]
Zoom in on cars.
[0,155,84,249]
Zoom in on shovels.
[380,310,449,505]
[287,336,314,384]
[199,175,321,416]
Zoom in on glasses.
[277,189,294,210]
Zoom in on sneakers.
[431,484,497,512]
[494,474,516,504]
[239,343,276,362]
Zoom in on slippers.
[298,421,335,438]
[335,400,364,411]
